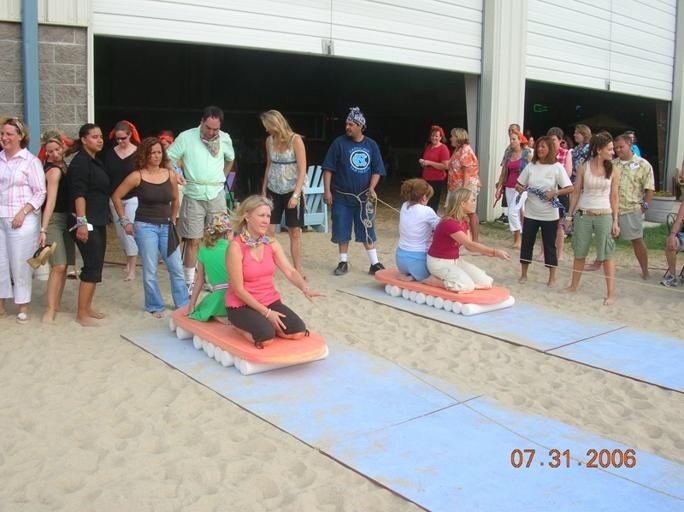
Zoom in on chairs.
[663,212,684,278]
[281,165,328,233]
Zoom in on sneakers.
[369,262,385,274]
[495,213,509,226]
[334,263,348,275]
[201,280,214,292]
[661,273,678,286]
[187,283,194,298]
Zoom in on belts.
[578,209,612,215]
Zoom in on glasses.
[116,135,129,140]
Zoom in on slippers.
[16,312,30,323]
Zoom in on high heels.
[26,242,57,268]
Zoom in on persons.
[183,194,326,348]
[321,107,386,275]
[495,123,683,304]
[393,125,511,295]
[0,104,234,327]
[259,105,306,277]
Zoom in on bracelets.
[293,194,301,199]
